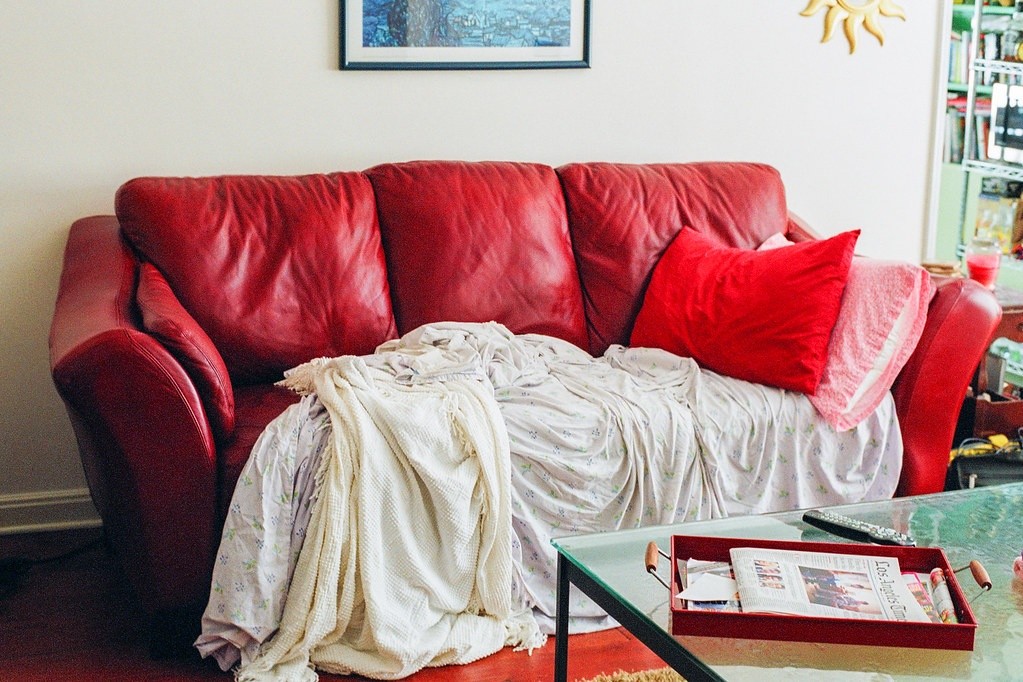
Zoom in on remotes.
[802,509,916,548]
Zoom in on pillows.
[630,226,863,391]
[757,232,936,433]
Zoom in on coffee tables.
[553,482,1023,682]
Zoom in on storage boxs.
[645,531,994,651]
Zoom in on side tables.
[972,284,1022,438]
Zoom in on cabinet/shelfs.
[935,0,1023,272]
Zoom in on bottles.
[967,236,1001,291]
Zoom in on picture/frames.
[336,0,592,70]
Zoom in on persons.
[756,561,784,590]
[803,571,870,607]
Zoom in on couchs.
[48,159,1004,682]
